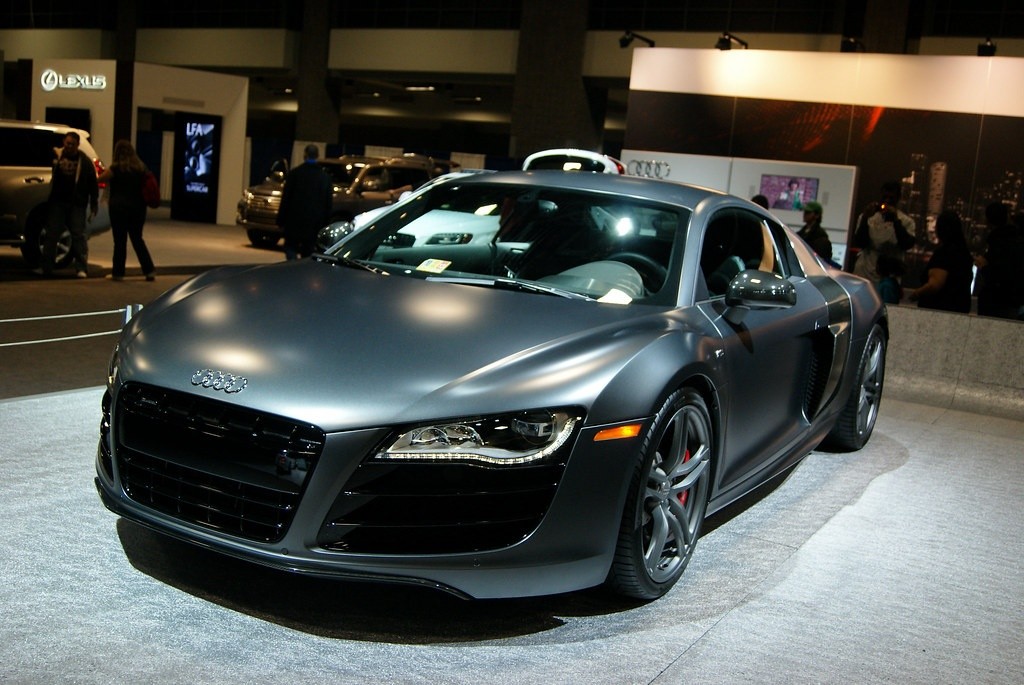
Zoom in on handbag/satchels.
[143,171,160,208]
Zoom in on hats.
[802,201,822,218]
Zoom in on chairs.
[514,206,608,280]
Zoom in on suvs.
[237,153,471,254]
[0,120,113,267]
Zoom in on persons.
[275,145,335,260]
[34,132,100,279]
[779,179,803,209]
[751,181,1024,322]
[96,140,157,280]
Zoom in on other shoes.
[77,271,87,277]
[32,268,44,275]
[106,274,121,281]
[146,275,155,281]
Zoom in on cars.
[317,163,661,282]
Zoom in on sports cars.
[93,170,891,625]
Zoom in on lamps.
[714,30,748,50]
[840,38,866,52]
[978,34,996,56]
[619,31,655,49]
